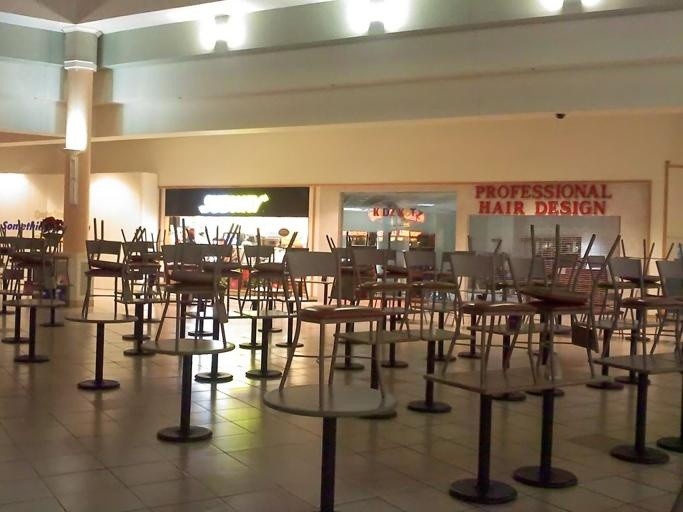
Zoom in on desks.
[232,290,268,352]
[514,359,596,490]
[585,313,639,389]
[387,272,415,321]
[0,268,20,316]
[1,289,31,345]
[64,308,144,392]
[8,294,67,363]
[470,318,530,402]
[138,270,166,322]
[433,363,527,503]
[656,347,683,453]
[323,282,371,370]
[260,381,399,512]
[38,272,72,327]
[457,290,496,359]
[395,326,474,412]
[187,305,240,383]
[274,290,314,346]
[333,325,417,420]
[517,314,569,398]
[423,292,458,362]
[374,286,415,368]
[142,337,236,442]
[613,310,661,386]
[236,303,299,379]
[183,280,216,338]
[595,345,678,467]
[115,290,161,356]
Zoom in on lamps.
[62,148,84,205]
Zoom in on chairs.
[123,231,162,270]
[77,237,131,319]
[7,249,60,306]
[334,249,374,306]
[403,240,460,337]
[624,257,667,323]
[246,244,289,318]
[155,243,229,349]
[625,253,683,359]
[252,229,300,308]
[270,246,309,300]
[376,228,411,270]
[193,222,237,270]
[200,255,240,316]
[426,244,463,305]
[348,243,409,342]
[277,247,388,406]
[9,221,36,266]
[384,231,415,273]
[439,251,539,390]
[13,223,66,259]
[41,225,74,284]
[464,240,499,295]
[8,247,42,299]
[85,218,138,312]
[242,249,270,300]
[519,220,617,355]
[582,254,637,325]
[327,233,372,281]
[127,249,158,291]
